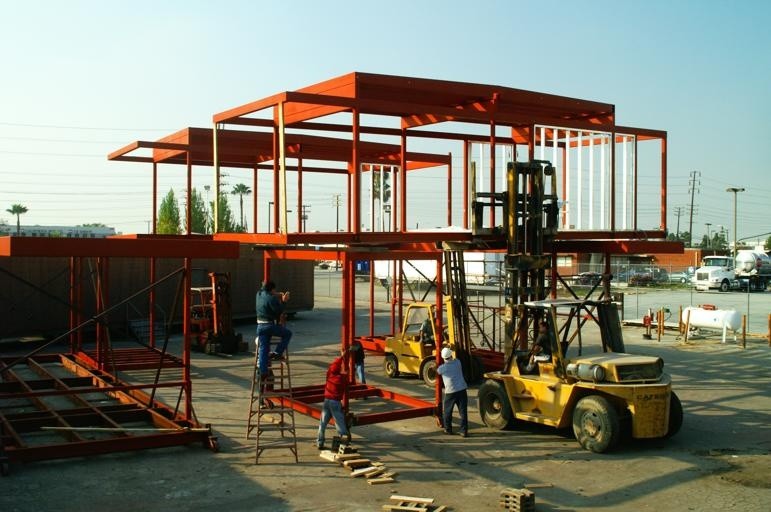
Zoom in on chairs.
[543,341,568,362]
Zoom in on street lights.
[727,188,745,268]
[268,201,274,234]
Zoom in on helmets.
[440,347,452,358]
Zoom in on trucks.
[695,250,770,292]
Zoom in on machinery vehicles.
[190,272,247,356]
[478,161,682,454]
[385,239,484,388]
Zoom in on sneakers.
[442,429,452,435]
[268,351,286,362]
[455,431,467,437]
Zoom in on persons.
[420,310,442,345]
[256,279,293,407]
[349,340,366,384]
[317,349,352,448]
[523,320,551,373]
[437,347,469,437]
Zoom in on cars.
[667,271,688,284]
[572,271,602,284]
